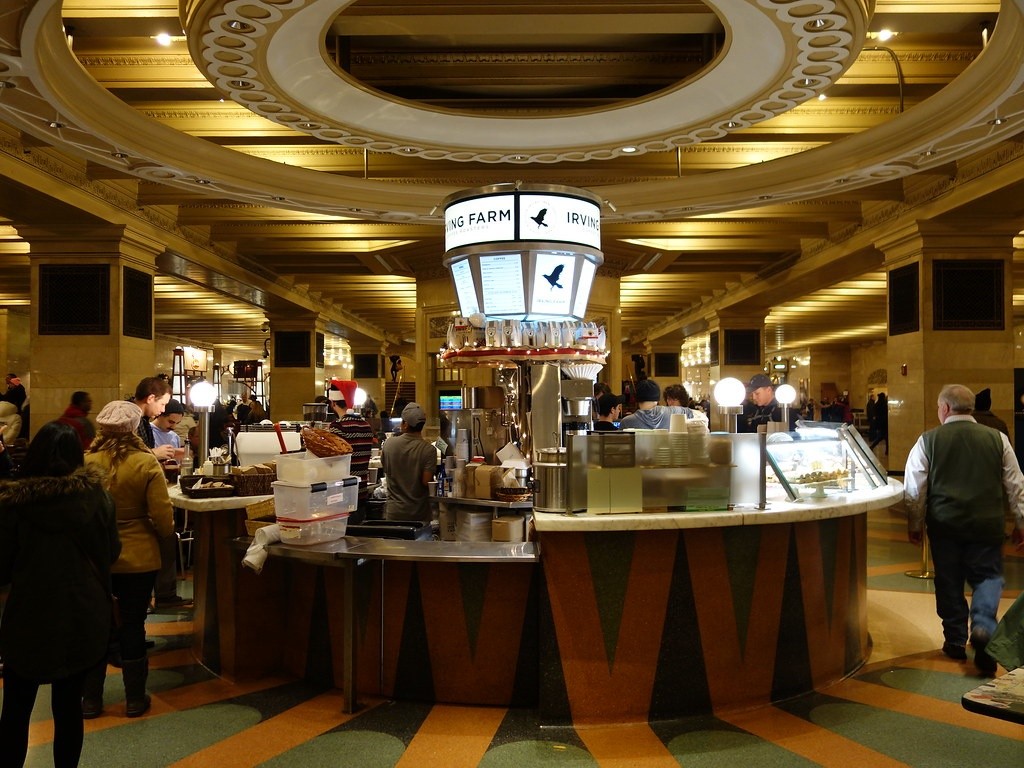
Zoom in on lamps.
[190,381,217,405]
[714,377,747,407]
[353,388,368,405]
[774,385,796,404]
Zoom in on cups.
[456,429,469,468]
[255,524,281,545]
[653,429,671,466]
[244,549,268,570]
[445,456,454,469]
[669,414,690,466]
[368,468,378,483]
[638,430,656,469]
[371,449,379,458]
[428,482,437,496]
[213,464,230,475]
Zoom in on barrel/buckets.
[531,462,586,512]
[536,447,567,462]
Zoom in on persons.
[631,354,647,381]
[799,395,854,425]
[126,377,270,615]
[0,419,122,768]
[390,355,401,382]
[592,383,625,431]
[866,392,888,455]
[902,383,1024,673]
[745,374,799,432]
[688,397,710,409]
[971,388,1009,435]
[619,380,696,430]
[0,374,30,480]
[328,380,373,537]
[81,400,174,719]
[59,390,96,453]
[379,411,394,432]
[315,395,338,422]
[381,402,437,521]
[664,384,689,407]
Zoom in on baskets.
[227,473,277,496]
[185,484,235,499]
[299,425,352,457]
[180,475,228,494]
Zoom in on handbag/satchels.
[111,593,122,629]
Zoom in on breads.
[232,460,277,474]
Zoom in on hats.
[96,400,142,433]
[745,373,771,393]
[329,379,358,415]
[974,388,992,412]
[403,402,427,426]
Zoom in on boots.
[117,655,150,717]
[81,661,108,717]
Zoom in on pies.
[201,482,224,488]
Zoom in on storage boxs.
[438,464,533,542]
[269,450,361,546]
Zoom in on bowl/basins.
[271,452,361,545]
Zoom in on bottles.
[203,458,213,475]
[181,439,193,476]
[710,431,733,464]
[436,463,446,497]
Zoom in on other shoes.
[970,627,997,677]
[147,639,154,649]
[106,644,123,667]
[155,595,182,613]
[942,641,968,663]
[146,605,155,614]
